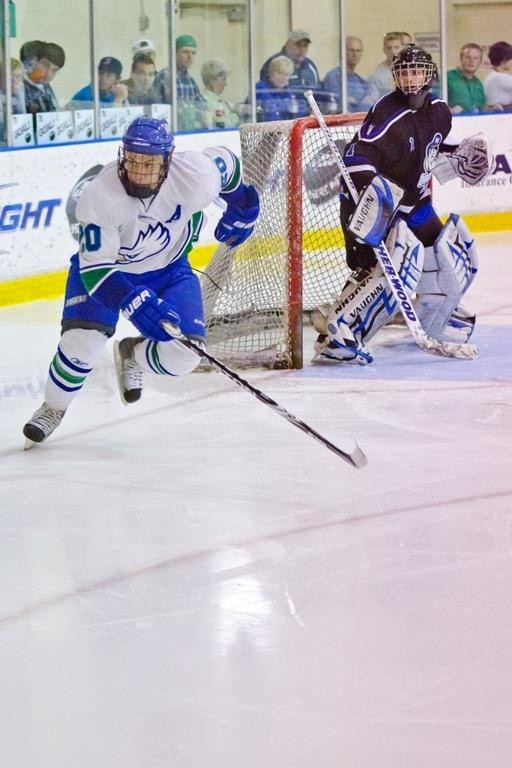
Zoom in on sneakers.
[119,336,145,403]
[23,402,66,442]
[314,334,354,360]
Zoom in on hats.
[100,57,122,77]
[131,39,154,55]
[287,28,312,43]
[177,35,195,48]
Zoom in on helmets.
[117,116,175,198]
[392,46,435,95]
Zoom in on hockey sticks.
[301,91,477,361]
[163,320,366,468]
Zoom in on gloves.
[214,185,260,250]
[118,284,180,342]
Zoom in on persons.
[125,35,207,111]
[447,43,504,113]
[259,28,319,100]
[242,56,298,122]
[11,39,64,114]
[23,113,261,443]
[483,41,511,105]
[74,56,128,107]
[311,41,495,361]
[368,32,413,100]
[201,58,240,126]
[322,35,371,113]
